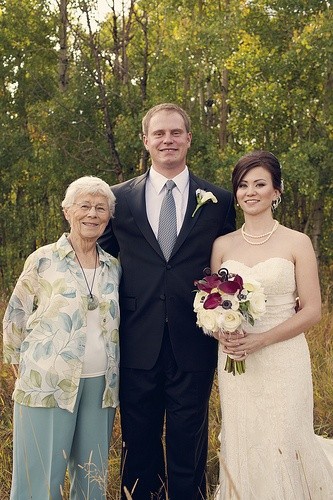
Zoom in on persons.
[96,103,236,500]
[2,176,123,500]
[211,151,333,500]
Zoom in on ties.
[157,180,177,262]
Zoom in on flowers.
[192,188,218,217]
[192,260,267,376]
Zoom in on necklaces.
[241,220,279,245]
[68,236,99,310]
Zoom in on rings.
[244,349,248,357]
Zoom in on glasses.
[73,201,110,213]
